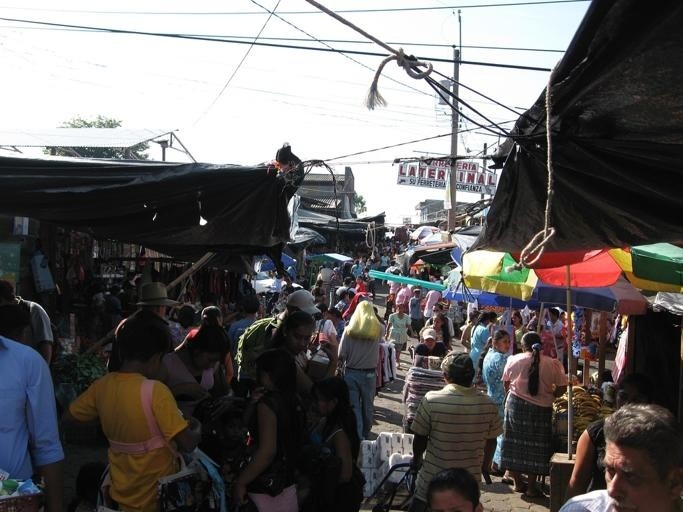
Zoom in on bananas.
[552,385,615,436]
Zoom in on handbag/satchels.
[301,319,331,381]
[230,390,290,498]
[154,437,226,511]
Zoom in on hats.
[285,289,322,315]
[421,327,437,342]
[133,281,181,307]
[440,351,475,379]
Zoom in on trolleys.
[363,461,418,512]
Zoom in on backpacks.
[235,313,280,377]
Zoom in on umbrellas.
[461,241,683,460]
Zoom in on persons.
[365,268,377,300]
[100,283,178,348]
[313,279,325,287]
[426,467,484,512]
[512,310,526,352]
[342,262,356,280]
[160,326,230,412]
[500,332,568,498]
[395,281,410,313]
[488,312,498,333]
[103,286,123,311]
[380,252,391,272]
[308,304,338,382]
[333,277,355,300]
[460,310,479,352]
[558,403,683,512]
[225,294,260,344]
[119,271,136,288]
[207,396,251,480]
[270,288,321,340]
[311,288,327,308]
[409,288,422,338]
[0,336,65,512]
[313,377,367,512]
[424,303,454,351]
[411,350,503,512]
[339,300,384,441]
[565,373,656,497]
[332,291,349,312]
[174,306,234,395]
[315,262,332,303]
[478,330,516,484]
[470,312,491,390]
[270,310,317,359]
[420,313,447,343]
[65,312,195,510]
[235,349,304,512]
[386,270,401,298]
[422,289,442,318]
[356,276,365,293]
[415,329,446,356]
[549,308,566,364]
[384,303,416,368]
[351,260,362,276]
[1,279,53,367]
[330,267,341,281]
